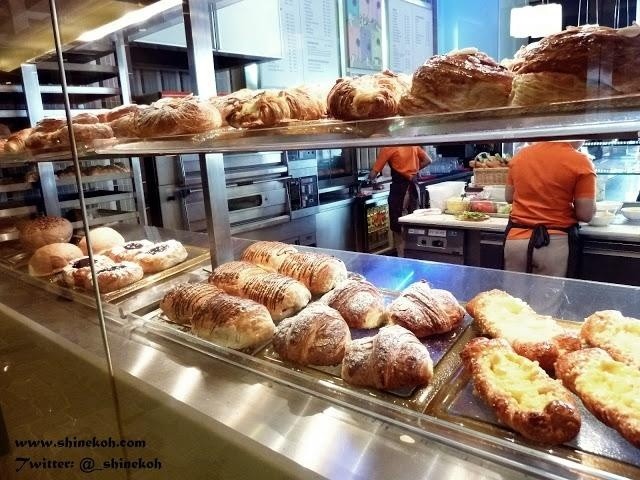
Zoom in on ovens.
[144,151,321,234]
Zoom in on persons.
[369,145,433,256]
[500,141,598,318]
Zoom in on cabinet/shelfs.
[0,95,640,480]
[0,40,148,242]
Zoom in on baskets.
[473,152,509,185]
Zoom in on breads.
[459,336,581,444]
[508,19,640,105]
[110,238,153,264]
[241,271,311,321]
[278,252,348,296]
[240,240,298,271]
[61,254,113,289]
[159,281,225,325]
[189,294,276,351]
[397,46,512,115]
[553,347,640,450]
[29,242,84,279]
[465,288,582,368]
[0,97,223,156]
[341,325,434,392]
[224,88,326,129]
[86,260,144,292]
[578,310,640,367]
[208,261,276,298]
[329,279,386,329]
[135,239,188,274]
[384,279,465,337]
[273,300,351,367]
[19,216,72,252]
[328,70,410,118]
[80,226,122,257]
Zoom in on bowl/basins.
[589,201,639,226]
